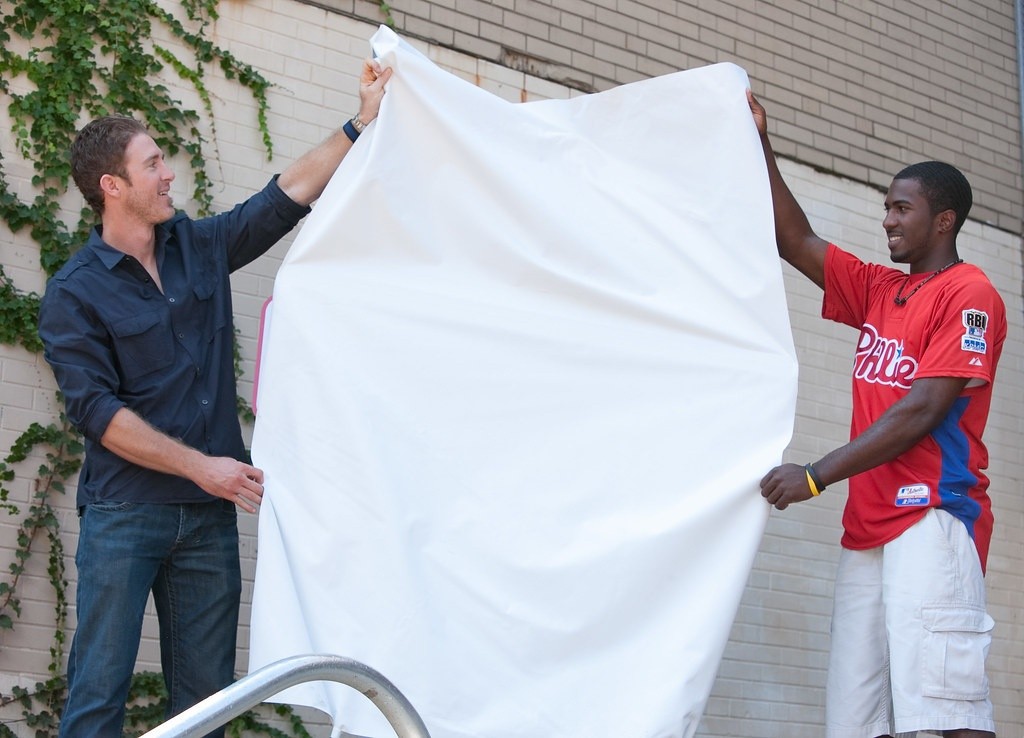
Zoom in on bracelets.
[349,114,367,133]
[805,463,825,499]
[342,120,360,143]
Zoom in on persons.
[38,56,394,738]
[744,83,1007,738]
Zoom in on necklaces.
[892,258,964,306]
